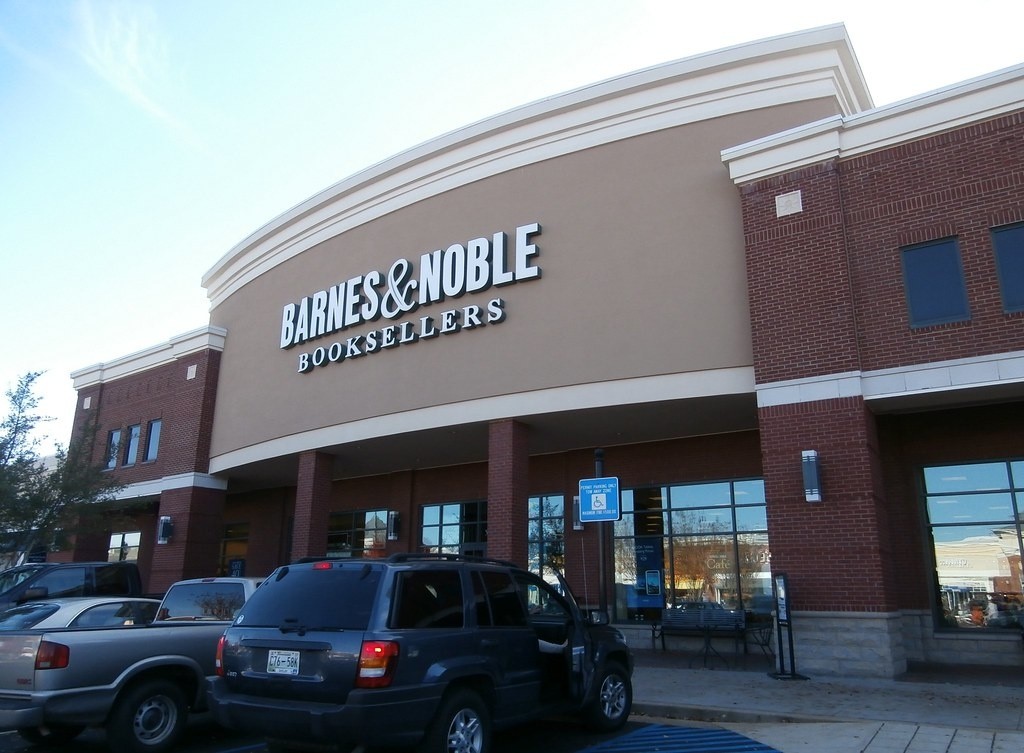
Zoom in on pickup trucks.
[1,576,269,750]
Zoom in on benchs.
[651,608,747,657]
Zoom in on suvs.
[208,549,634,753]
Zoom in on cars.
[0,560,138,614]
[0,596,163,630]
[672,601,724,611]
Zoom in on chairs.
[744,616,775,665]
